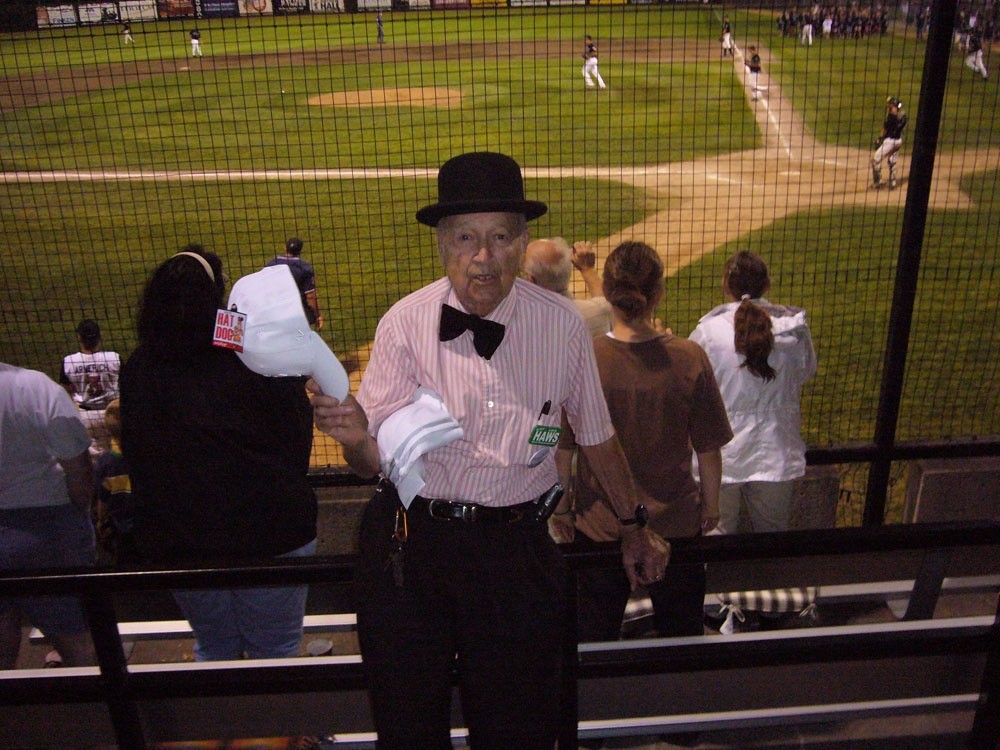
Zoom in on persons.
[964,29,989,81]
[580,35,607,90]
[721,14,734,57]
[0,363,100,668]
[524,237,612,339]
[273,244,323,331]
[121,23,136,45]
[549,239,734,643]
[59,320,125,450]
[104,245,318,664]
[777,0,889,47]
[376,10,386,44]
[870,96,907,190]
[686,249,816,534]
[744,46,770,102]
[190,27,203,57]
[305,151,670,750]
[901,0,1000,52]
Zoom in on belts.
[379,478,535,524]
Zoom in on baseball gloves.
[718,37,724,42]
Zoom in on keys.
[383,544,406,574]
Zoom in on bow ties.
[440,304,505,360]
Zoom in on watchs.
[620,504,649,527]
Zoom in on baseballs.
[282,90,285,93]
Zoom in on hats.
[416,152,547,228]
[227,264,350,406]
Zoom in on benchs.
[0,502,1000,750]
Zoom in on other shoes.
[868,180,882,190]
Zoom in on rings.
[653,574,658,580]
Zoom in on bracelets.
[553,509,572,516]
